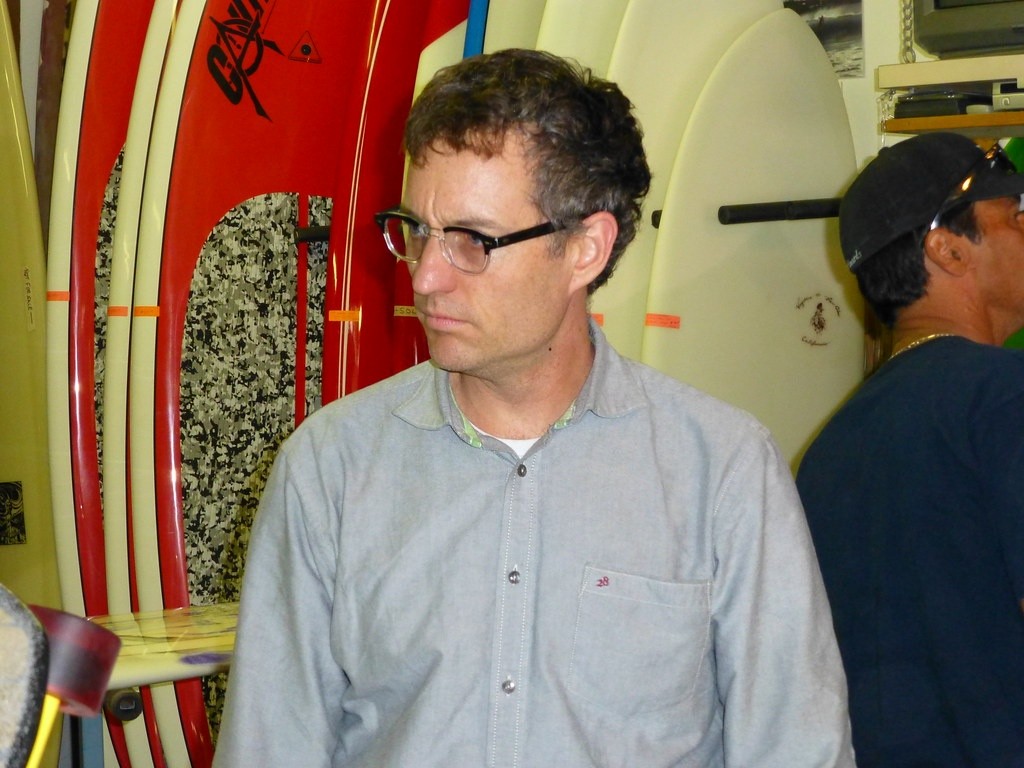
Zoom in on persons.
[210,48,858,768]
[794,129,1024,768]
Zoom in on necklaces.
[889,333,954,361]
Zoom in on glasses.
[374,207,621,276]
[919,144,1017,259]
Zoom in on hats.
[839,135,1024,274]
